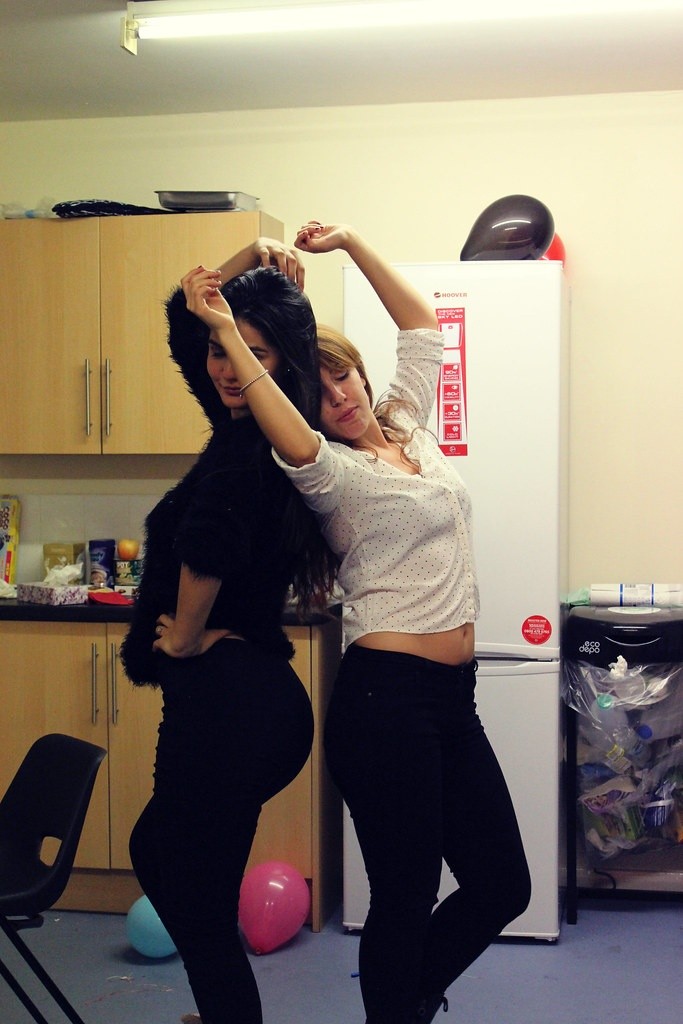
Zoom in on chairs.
[0,731,111,1024]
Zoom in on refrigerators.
[339,252,565,946]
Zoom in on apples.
[117,538,139,560]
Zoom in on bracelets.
[238,369,269,399]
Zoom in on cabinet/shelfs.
[3,209,286,458]
[0,618,340,935]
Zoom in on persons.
[117,234,323,1023]
[180,222,535,1024]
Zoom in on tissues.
[15,562,90,607]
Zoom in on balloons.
[536,233,566,266]
[459,193,556,261]
[125,893,178,958]
[237,859,311,955]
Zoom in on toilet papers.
[588,580,683,611]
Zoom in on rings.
[157,626,166,636]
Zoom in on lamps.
[116,0,683,58]
